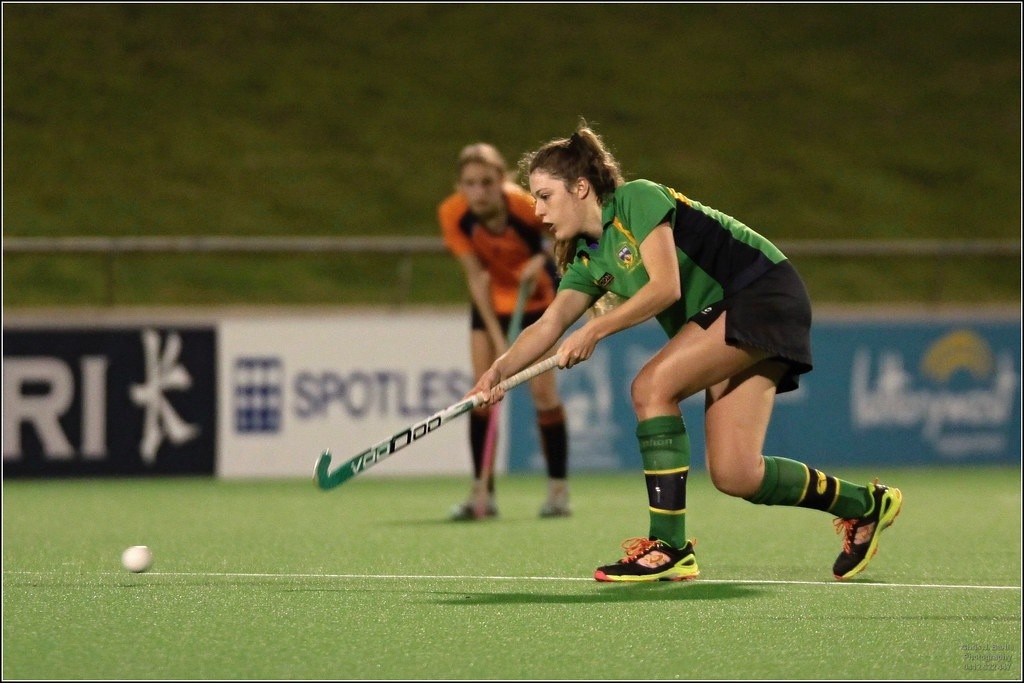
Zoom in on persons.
[463,128,902,583]
[434,140,574,521]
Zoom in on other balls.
[122,546,153,573]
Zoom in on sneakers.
[539,489,572,517]
[454,490,496,522]
[593,537,701,582]
[832,478,902,581]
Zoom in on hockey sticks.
[312,353,561,496]
